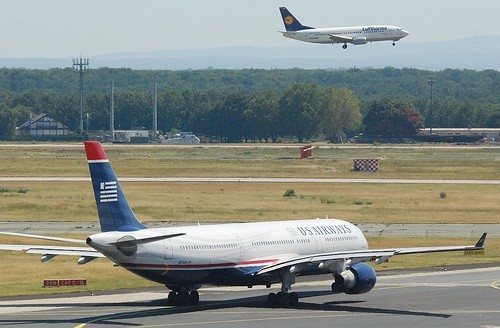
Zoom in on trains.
[419,128,500,142]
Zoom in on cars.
[168,134,200,145]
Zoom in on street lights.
[427,76,436,134]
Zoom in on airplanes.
[0,139,487,308]
[278,6,409,49]
